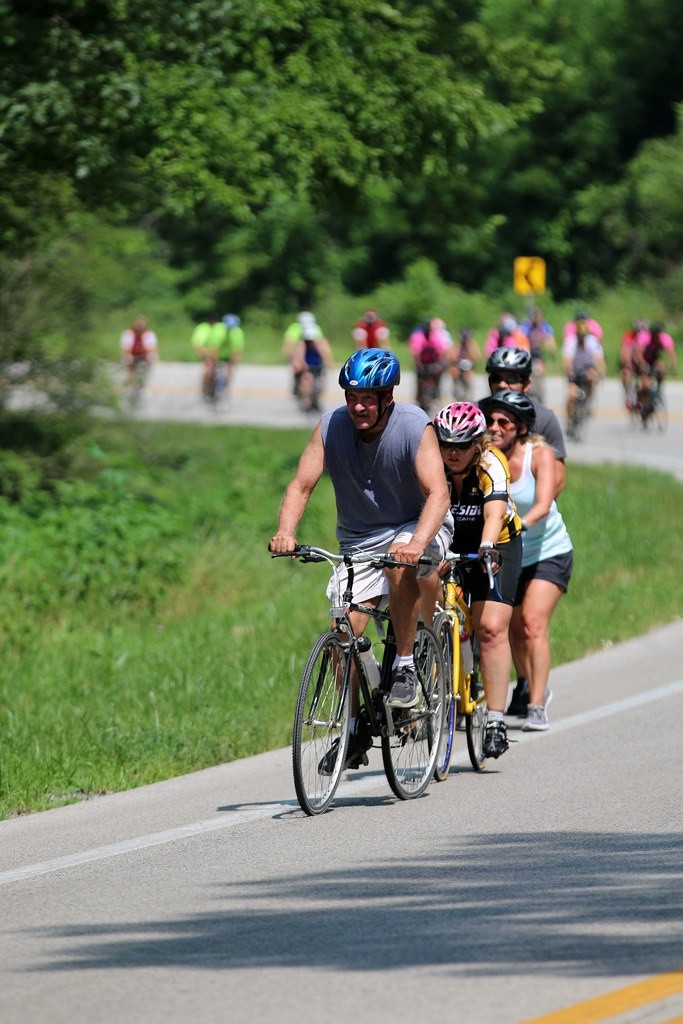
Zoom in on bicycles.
[628,365,670,429]
[268,543,494,814]
[569,370,594,435]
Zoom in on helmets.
[224,315,240,329]
[485,346,532,378]
[434,402,486,445]
[298,310,316,329]
[338,348,400,392]
[486,390,536,429]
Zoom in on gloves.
[478,545,504,566]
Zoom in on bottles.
[457,609,464,634]
[357,635,381,691]
[459,629,473,675]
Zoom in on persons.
[349,309,387,349]
[194,314,245,400]
[478,390,574,729]
[561,309,605,440]
[283,310,334,410]
[270,348,451,777]
[470,347,567,718]
[414,401,523,758]
[485,307,557,402]
[122,316,155,381]
[619,317,678,411]
[409,317,483,411]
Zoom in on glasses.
[440,442,473,450]
[491,375,526,384]
[486,416,518,426]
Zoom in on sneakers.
[506,678,531,715]
[543,686,553,706]
[482,720,519,759]
[386,664,423,708]
[318,730,374,776]
[523,704,550,731]
